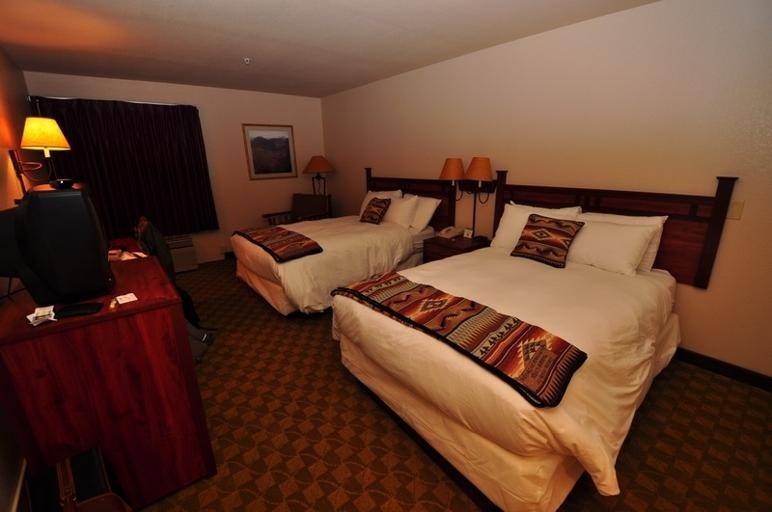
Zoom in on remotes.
[54,302,101,320]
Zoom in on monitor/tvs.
[0,186,115,307]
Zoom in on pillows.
[567,221,659,277]
[403,193,442,232]
[358,197,392,224]
[359,190,394,223]
[489,203,583,251]
[577,210,669,273]
[370,189,402,199]
[510,213,586,269]
[509,199,582,216]
[383,195,418,228]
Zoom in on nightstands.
[423,236,492,265]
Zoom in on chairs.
[262,193,332,225]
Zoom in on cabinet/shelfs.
[1,237,218,510]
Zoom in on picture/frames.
[241,124,298,181]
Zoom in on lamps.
[438,158,465,226]
[302,155,337,195]
[465,157,492,238]
[20,117,72,185]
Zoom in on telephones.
[438,226,464,239]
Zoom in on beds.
[330,170,740,511]
[229,168,456,317]
